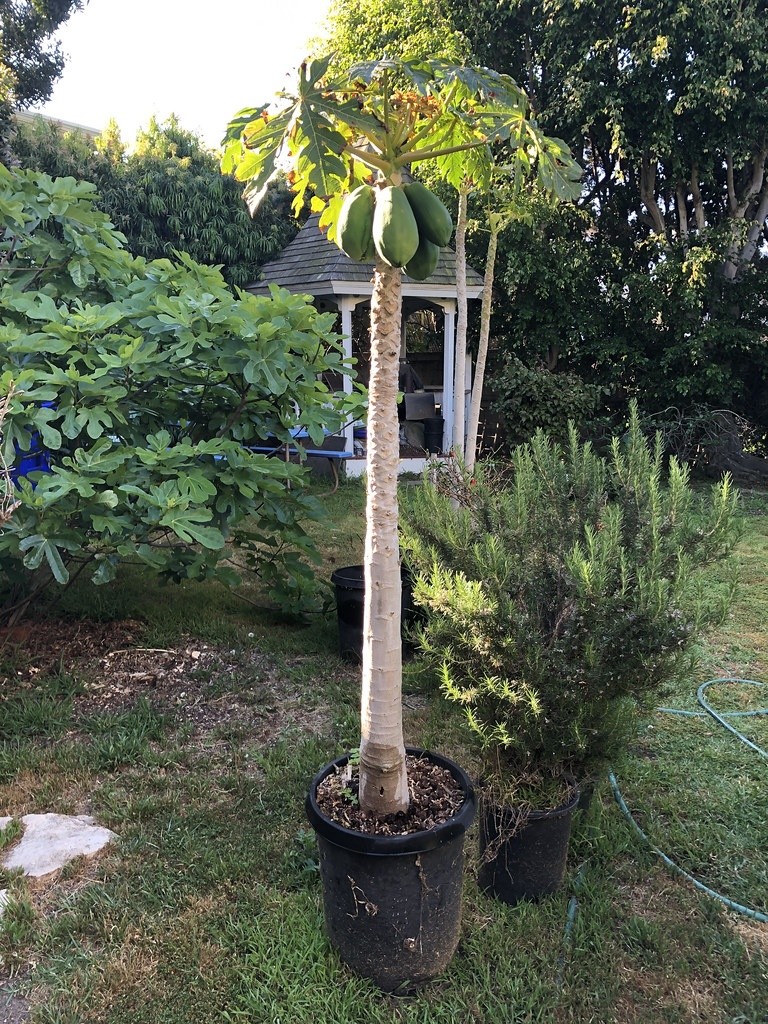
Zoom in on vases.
[330,564,416,663]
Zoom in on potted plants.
[221,58,581,994]
[401,392,744,901]
